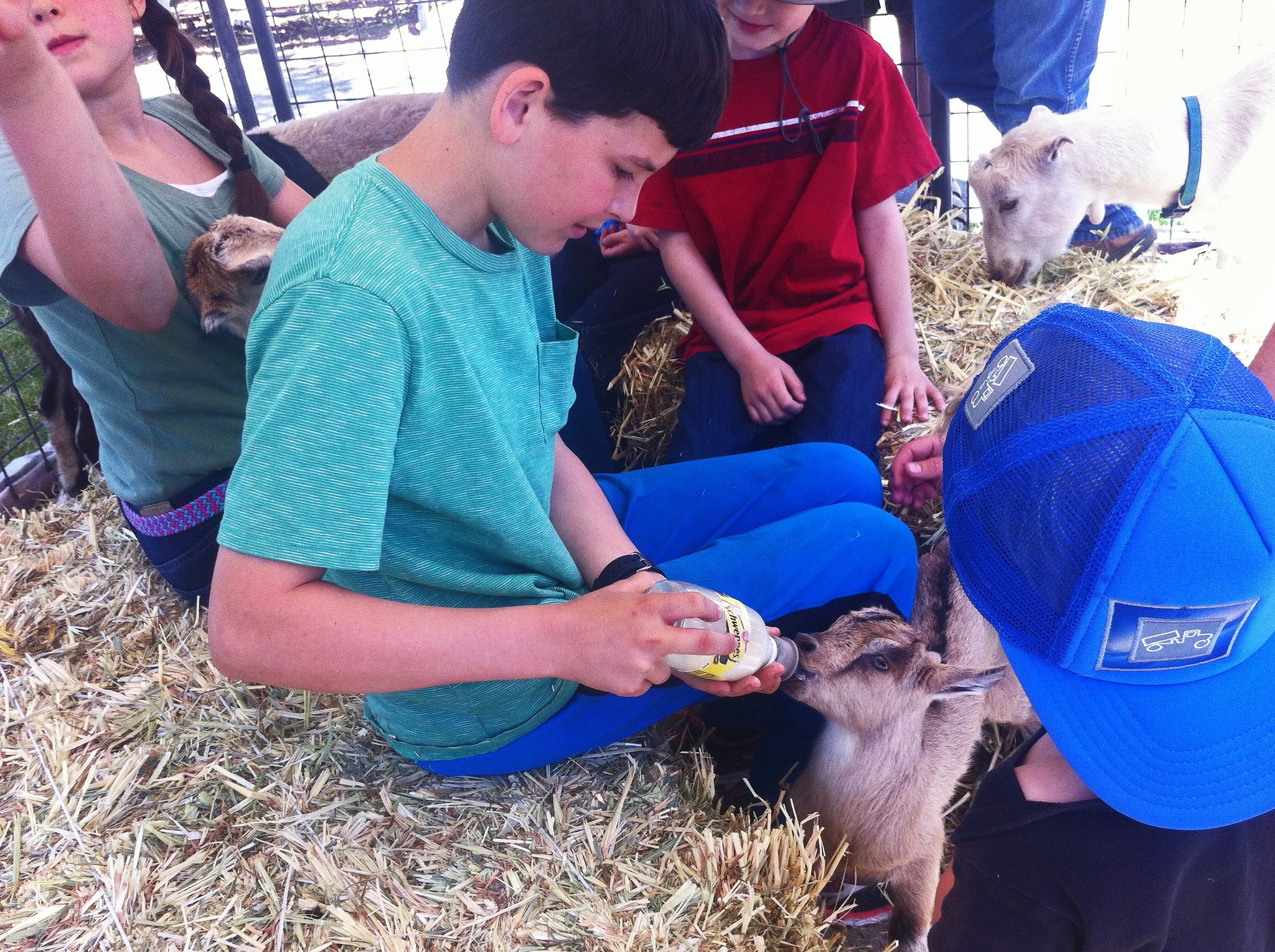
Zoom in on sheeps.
[11,92,442,498]
[969,40,1275,289]
[782,535,1039,951]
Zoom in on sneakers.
[820,886,897,926]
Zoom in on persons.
[455,0,1157,483]
[202,0,917,779]
[0,0,312,612]
[888,303,1275,952]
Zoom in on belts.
[120,481,226,538]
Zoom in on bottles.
[641,579,806,684]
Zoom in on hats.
[941,302,1275,830]
